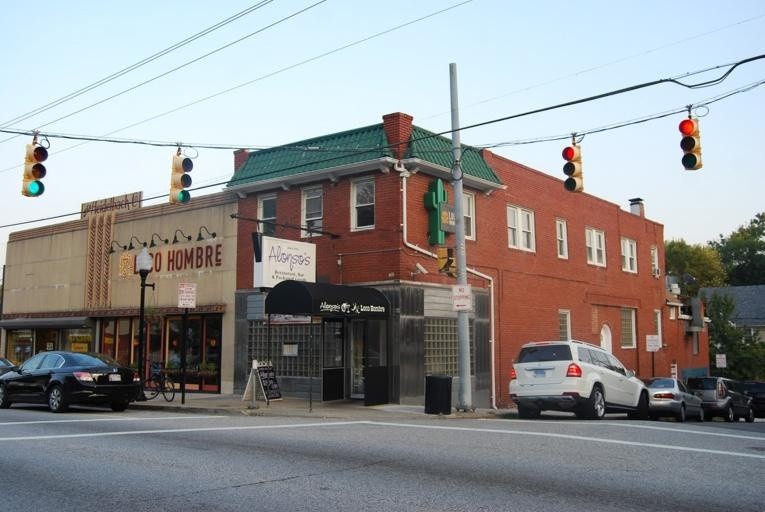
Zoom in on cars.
[641,375,708,423]
[0,350,139,413]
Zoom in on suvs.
[507,339,652,421]
[686,374,757,423]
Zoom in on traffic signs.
[451,283,473,312]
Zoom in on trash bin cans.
[425,375,452,414]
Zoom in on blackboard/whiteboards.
[257,366,281,399]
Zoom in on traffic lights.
[168,154,193,207]
[21,143,49,198]
[561,144,584,193]
[677,117,703,171]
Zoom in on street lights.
[136,247,155,401]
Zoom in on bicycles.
[143,372,176,402]
